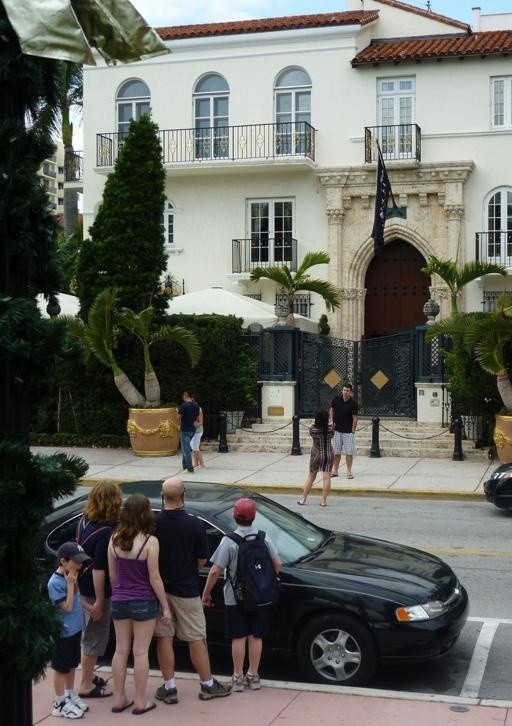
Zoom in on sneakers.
[52,699,84,719]
[246,673,259,690]
[155,685,178,704]
[231,674,245,692]
[64,692,88,713]
[199,679,232,698]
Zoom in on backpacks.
[225,530,280,614]
[75,516,111,580]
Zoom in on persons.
[203,499,281,692]
[107,495,172,715]
[47,543,94,719]
[330,384,357,479]
[153,479,231,705]
[297,411,334,505]
[191,394,205,470]
[176,391,201,474]
[76,479,122,699]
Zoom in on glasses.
[160,494,185,513]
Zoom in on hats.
[56,543,92,563]
[235,500,256,522]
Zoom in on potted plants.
[64,288,181,457]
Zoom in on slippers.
[78,684,112,698]
[132,698,155,715]
[111,697,133,711]
[297,501,305,504]
[319,503,326,506]
[346,474,354,477]
[91,676,108,685]
[330,473,339,478]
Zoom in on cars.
[34,478,471,686]
[483,461,512,509]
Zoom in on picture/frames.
[429,314,512,462]
